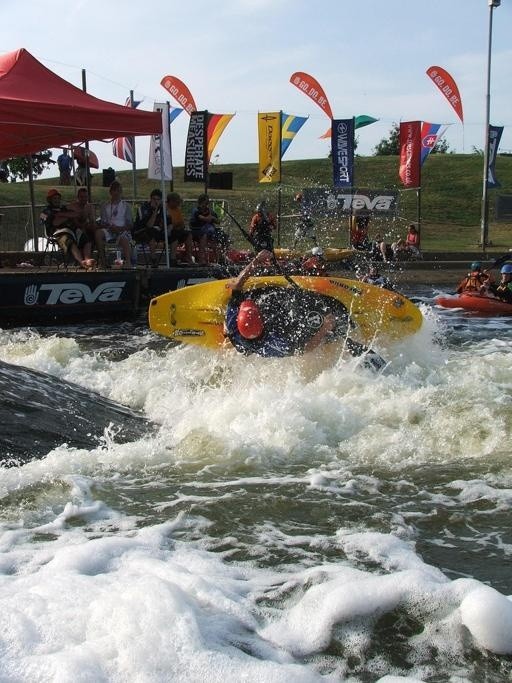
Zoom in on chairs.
[36,221,165,272]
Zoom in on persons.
[247,193,420,293]
[225,249,337,356]
[457,260,512,303]
[38,181,235,269]
[56,147,88,186]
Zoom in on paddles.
[448,254,511,295]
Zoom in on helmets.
[236,299,263,340]
[46,189,61,198]
[311,246,325,256]
[471,262,512,274]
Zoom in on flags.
[111,96,135,164]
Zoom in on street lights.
[478,0,501,246]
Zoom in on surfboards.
[219,205,386,373]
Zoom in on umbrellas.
[73,146,99,170]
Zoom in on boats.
[433,293,512,317]
[148,275,424,351]
